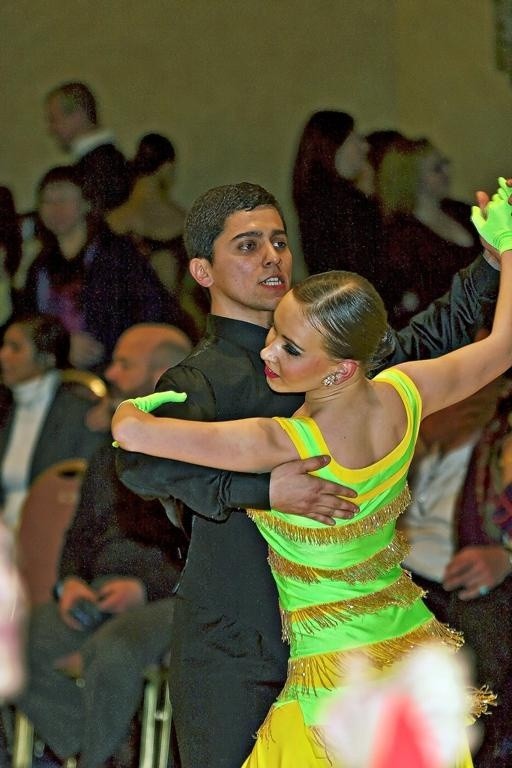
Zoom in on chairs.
[13,371,173,768]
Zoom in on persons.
[1,84,195,768]
[293,109,485,331]
[394,389,511,768]
